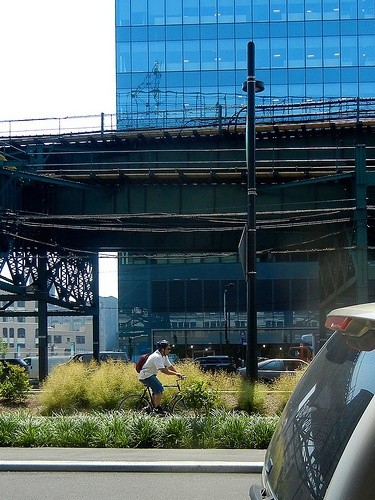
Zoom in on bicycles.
[117,375,210,422]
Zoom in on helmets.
[157,340,169,349]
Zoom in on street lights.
[241,42,265,383]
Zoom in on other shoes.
[144,406,149,413]
[155,406,167,414]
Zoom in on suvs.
[249,302,375,500]
[0,358,31,390]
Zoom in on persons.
[138,340,183,415]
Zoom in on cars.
[167,353,180,365]
[236,358,310,384]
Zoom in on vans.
[22,356,69,380]
[68,351,129,368]
[192,355,234,374]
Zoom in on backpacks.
[135,353,162,372]
